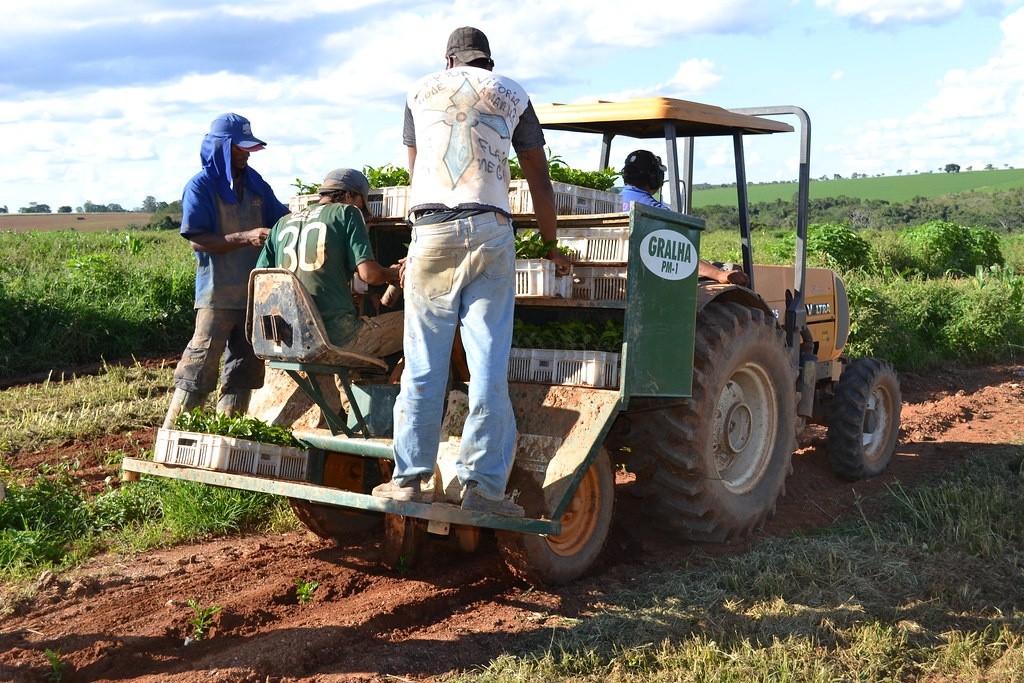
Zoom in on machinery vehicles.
[121,93,902,592]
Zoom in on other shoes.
[372,477,421,501]
[460,487,525,517]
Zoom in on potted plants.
[152,404,311,485]
[291,146,633,391]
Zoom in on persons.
[371,25,574,519]
[158,110,296,430]
[617,150,750,289]
[254,166,407,418]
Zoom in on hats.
[238,144,266,152]
[444,27,495,67]
[624,150,665,189]
[318,168,373,215]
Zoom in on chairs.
[244,267,390,439]
